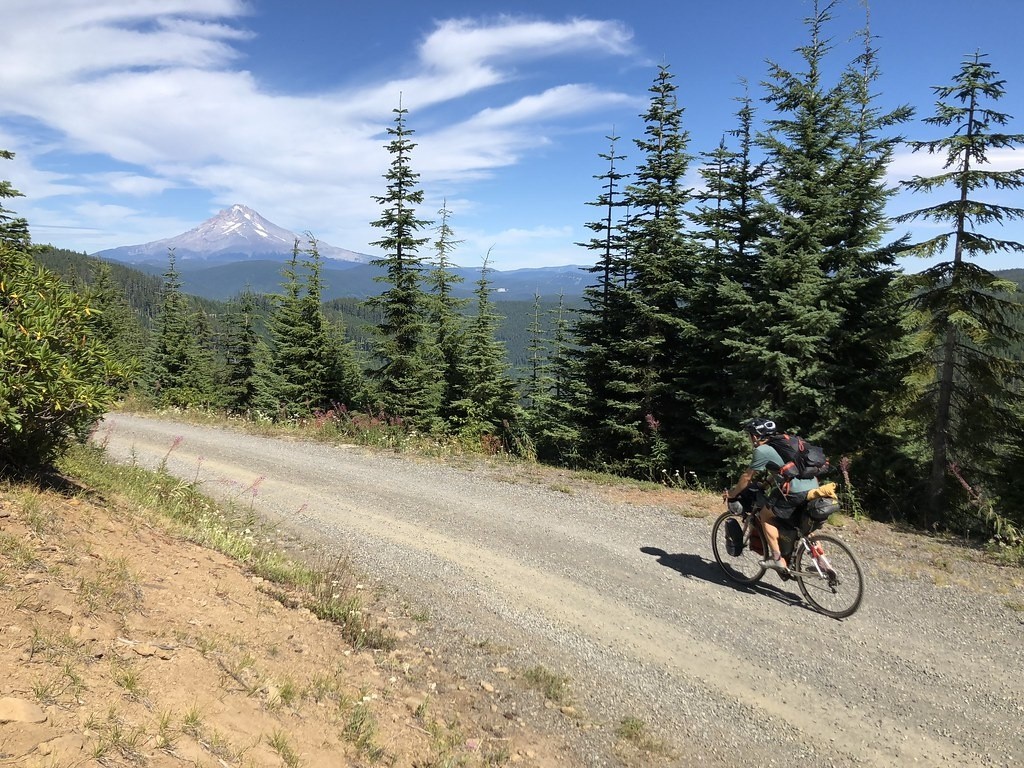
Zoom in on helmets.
[743,418,777,438]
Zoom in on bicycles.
[712,483,865,618]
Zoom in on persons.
[721,413,836,579]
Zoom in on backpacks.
[764,434,830,479]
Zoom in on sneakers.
[806,565,833,572]
[758,556,787,569]
